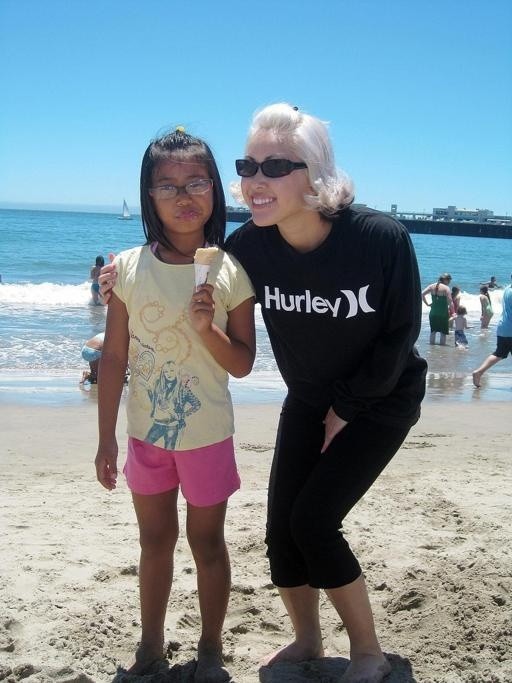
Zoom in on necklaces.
[155,244,194,264]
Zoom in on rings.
[98,280,106,287]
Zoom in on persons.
[95,104,429,682]
[479,276,503,289]
[90,255,104,306]
[95,127,256,680]
[78,330,131,384]
[422,273,474,347]
[480,286,494,328]
[472,274,512,387]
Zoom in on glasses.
[151,179,214,200]
[236,159,307,178]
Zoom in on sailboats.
[118,198,134,219]
